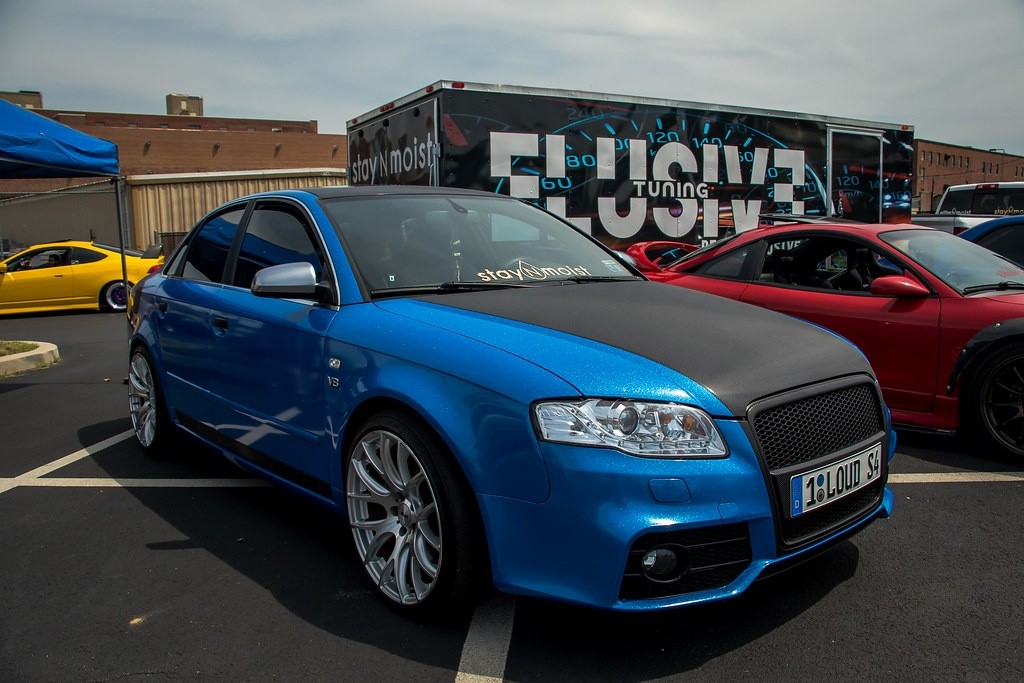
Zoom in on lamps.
[276,143,282,150]
[214,143,220,149]
[145,140,153,147]
[334,144,339,151]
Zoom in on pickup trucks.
[910,182,1024,236]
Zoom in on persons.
[859,249,903,287]
[839,190,852,219]
[17,253,59,270]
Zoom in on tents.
[0,96,128,304]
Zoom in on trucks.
[345,81,919,270]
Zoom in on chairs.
[982,198,997,209]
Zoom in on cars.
[0,239,166,316]
[625,223,1023,469]
[906,216,1024,289]
[123,185,898,624]
